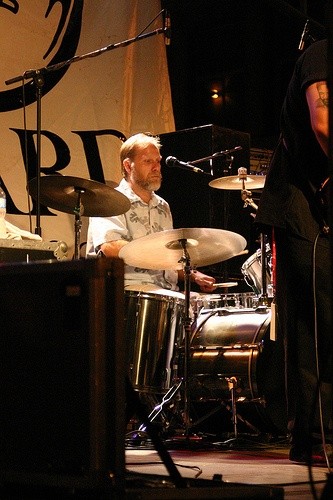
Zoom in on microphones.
[163,10,172,45]
[298,18,311,50]
[165,156,204,174]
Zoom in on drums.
[190,307,272,403]
[124,284,190,394]
[192,293,240,316]
[241,243,274,298]
[241,292,260,308]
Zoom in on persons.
[86,134,218,293]
[254,39,333,467]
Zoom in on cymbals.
[26,176,131,217]
[119,228,247,271]
[209,175,266,190]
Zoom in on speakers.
[0,256,125,500]
[155,123,251,292]
